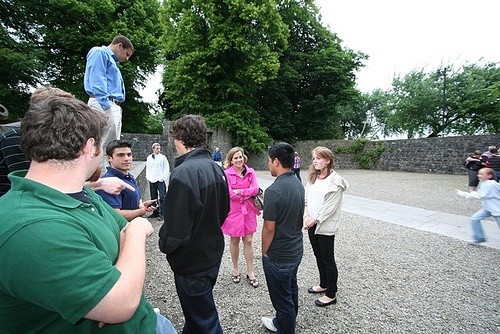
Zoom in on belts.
[109,98,121,105]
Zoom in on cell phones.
[147,201,158,209]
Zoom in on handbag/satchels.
[254,196,264,211]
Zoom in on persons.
[303,146,348,307]
[145,143,170,221]
[456,167,500,243]
[223,146,259,288]
[85,35,135,175]
[291,151,302,183]
[158,115,231,334]
[214,148,221,167]
[0,88,178,334]
[464,145,500,190]
[261,143,305,334]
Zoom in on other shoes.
[473,239,486,243]
[308,287,327,292]
[262,316,277,332]
[316,296,337,306]
[159,217,164,221]
[148,214,159,218]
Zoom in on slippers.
[232,272,240,283]
[247,273,258,288]
